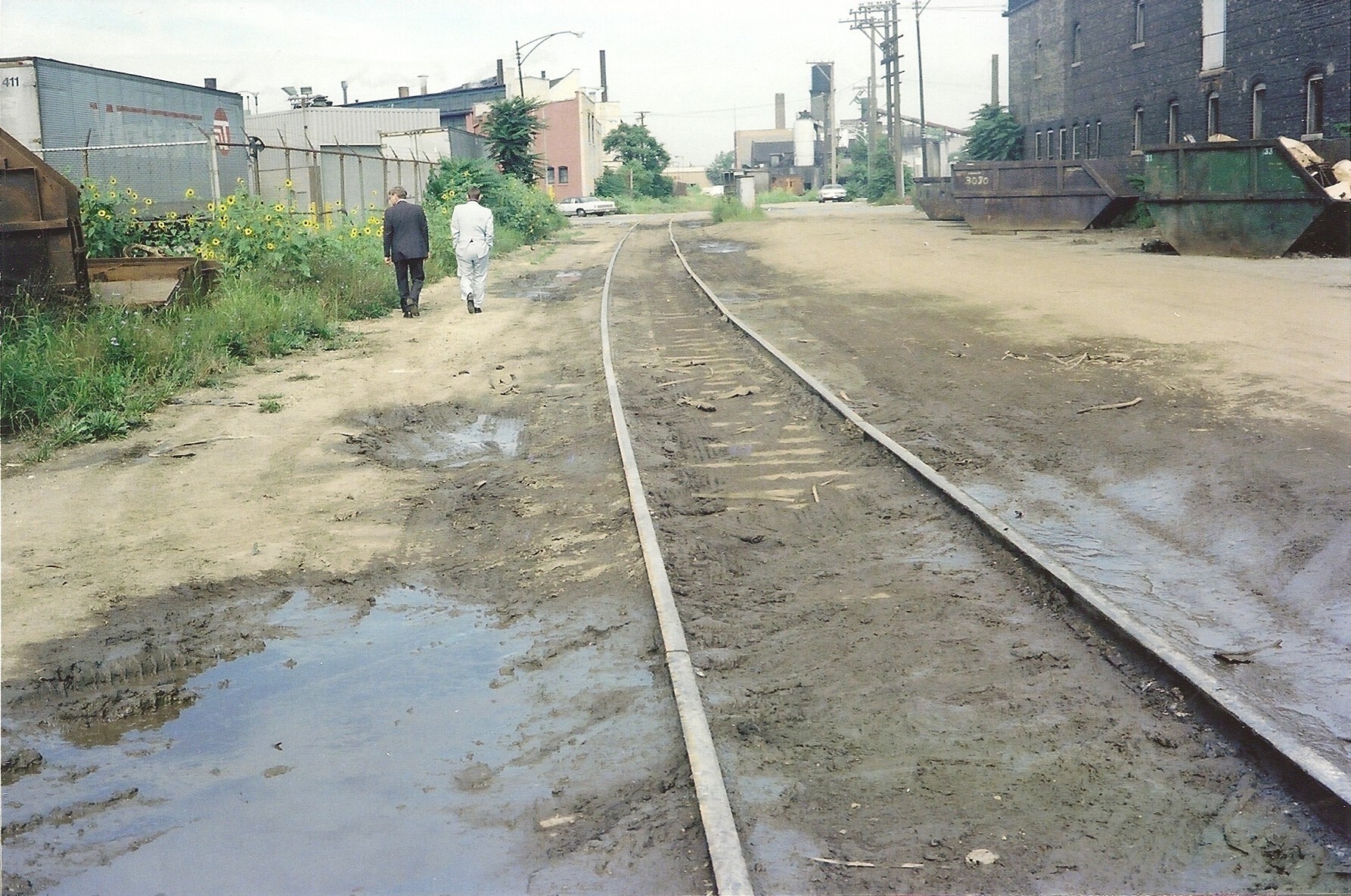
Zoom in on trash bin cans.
[912,177,965,222]
[1141,136,1351,258]
[950,157,1142,236]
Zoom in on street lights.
[516,31,584,98]
[236,90,259,113]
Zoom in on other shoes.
[467,293,475,314]
[474,307,482,313]
[406,298,420,316]
[403,311,412,318]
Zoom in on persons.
[383,186,429,318]
[451,187,494,313]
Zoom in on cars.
[819,181,847,203]
[555,196,616,217]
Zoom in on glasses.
[389,196,392,200]
